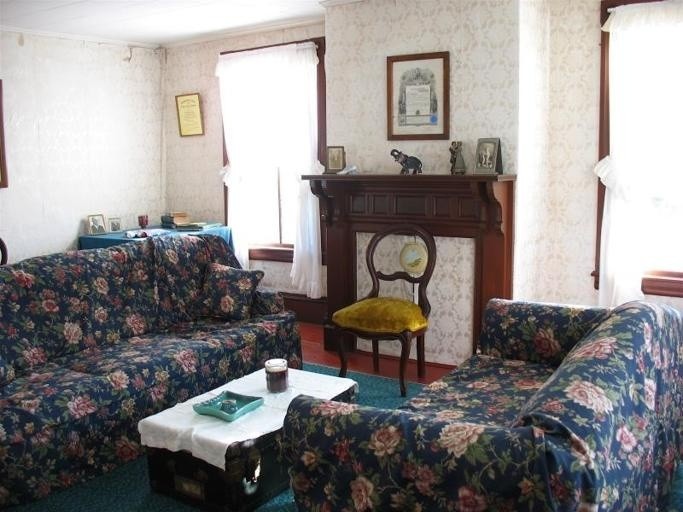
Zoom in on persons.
[449,141,457,176]
[479,145,493,168]
[89,216,104,234]
[454,140,465,175]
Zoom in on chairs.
[331,222,437,397]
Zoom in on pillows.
[196,262,265,321]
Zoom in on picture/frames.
[174,93,206,136]
[472,138,503,174]
[87,213,107,234]
[323,145,346,174]
[384,50,453,144]
[110,217,121,232]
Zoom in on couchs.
[0,231,303,508]
[283,296,683,512]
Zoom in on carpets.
[13,355,430,512]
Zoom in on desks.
[80,222,234,257]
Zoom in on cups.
[265,358,289,393]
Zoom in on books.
[160,210,222,231]
